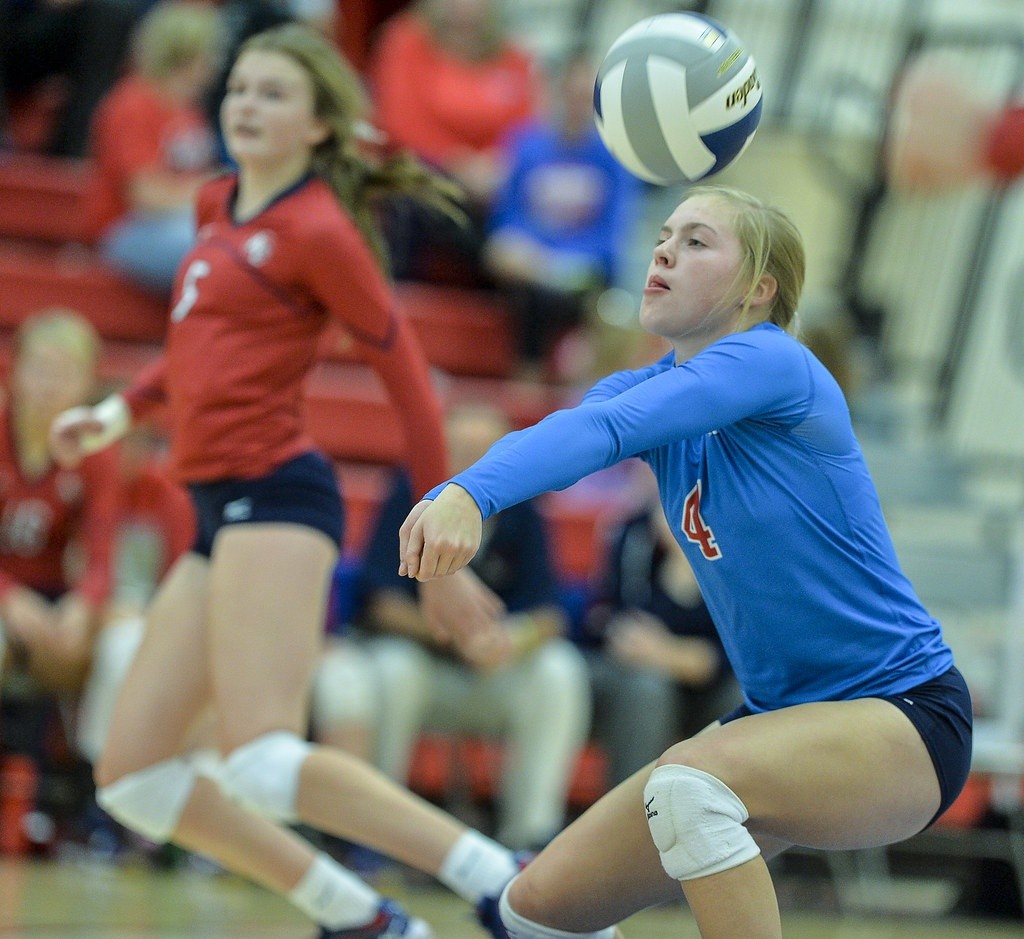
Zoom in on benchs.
[0,144,991,829]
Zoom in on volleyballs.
[593,10,764,188]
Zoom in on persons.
[397,187,972,939]
[46,22,534,939]
[0,0,1024,939]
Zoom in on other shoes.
[317,898,429,939]
[475,849,537,939]
